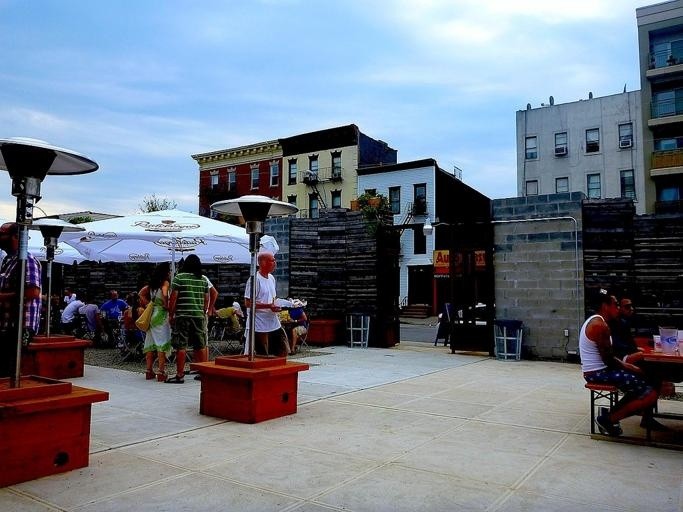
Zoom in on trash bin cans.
[494,319,523,362]
[345,307,375,348]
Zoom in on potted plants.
[350,191,394,235]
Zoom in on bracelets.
[294,319,298,323]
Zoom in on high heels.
[146,368,155,380]
[157,370,167,381]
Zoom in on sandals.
[165,374,185,384]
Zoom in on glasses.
[615,301,620,307]
[624,303,635,310]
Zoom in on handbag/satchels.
[134,301,154,332]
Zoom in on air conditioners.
[620,139,632,148]
[554,146,567,156]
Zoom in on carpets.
[83,347,328,375]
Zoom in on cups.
[659,328,679,355]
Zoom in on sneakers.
[290,347,296,355]
[640,417,667,431]
[595,415,622,435]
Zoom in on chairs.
[72,303,312,367]
[480,310,495,326]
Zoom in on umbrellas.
[55,207,279,292]
[0,228,86,336]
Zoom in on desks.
[451,323,495,356]
[632,337,683,453]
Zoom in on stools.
[584,382,620,438]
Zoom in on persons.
[41,254,244,382]
[578,295,655,434]
[287,307,308,355]
[242,252,290,357]
[0,221,42,377]
[607,296,671,432]
